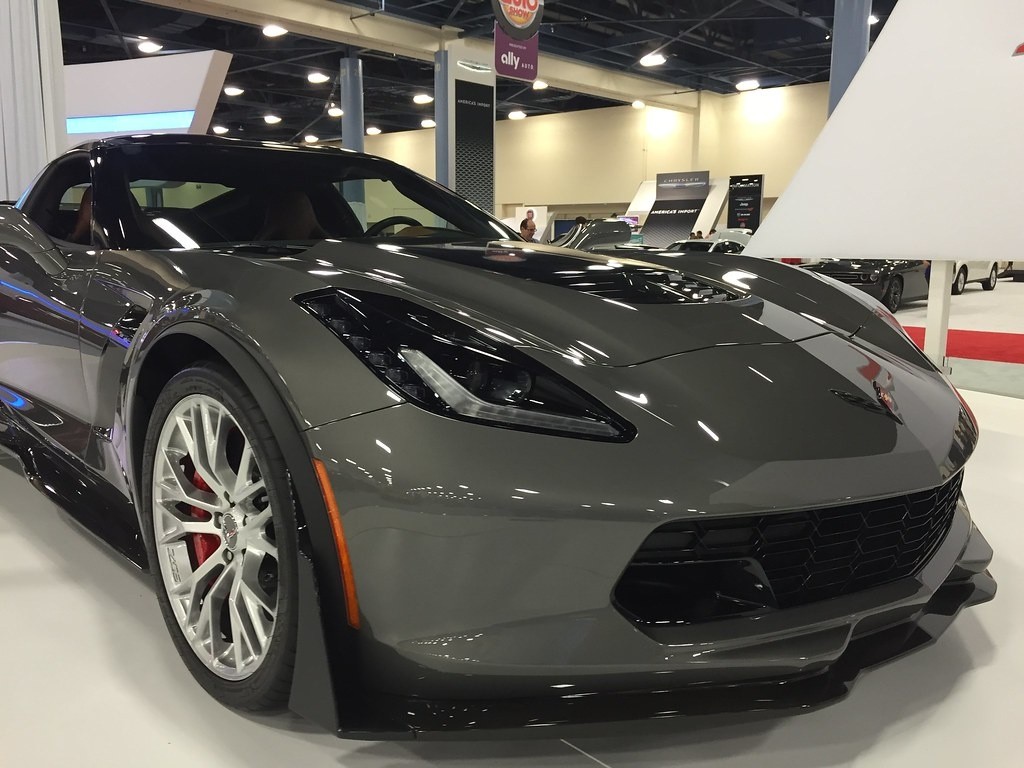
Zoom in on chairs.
[256,189,326,242]
[71,184,180,252]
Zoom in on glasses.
[526,229,537,232]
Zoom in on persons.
[697,231,703,239]
[520,219,539,243]
[611,212,618,218]
[690,233,698,239]
[709,229,716,235]
[574,216,586,226]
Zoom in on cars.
[665,228,753,256]
[796,259,930,314]
[953,260,1014,295]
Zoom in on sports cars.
[0,129,996,737]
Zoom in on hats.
[527,209,533,212]
[575,216,586,224]
